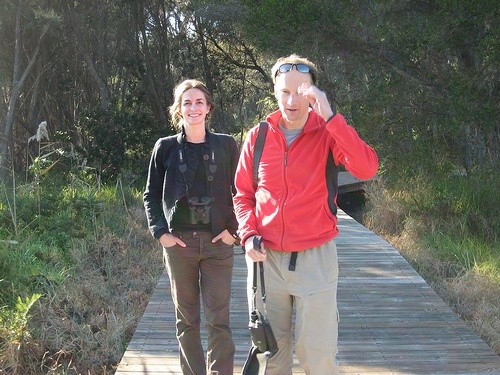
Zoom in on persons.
[143,79,241,375]
[233,55,378,375]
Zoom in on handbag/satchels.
[241,311,279,375]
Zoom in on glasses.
[275,63,315,78]
